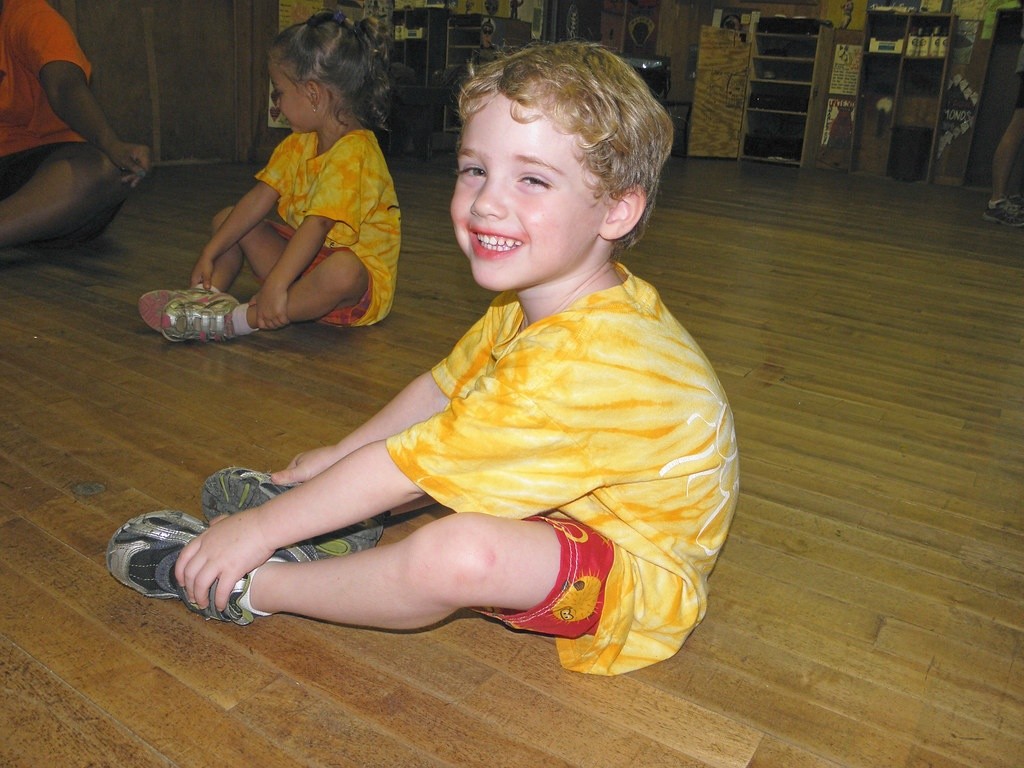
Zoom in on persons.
[0,0,154,248]
[982,31,1024,226]
[106,39,741,676]
[138,7,402,342]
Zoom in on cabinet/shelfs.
[684,16,839,170]
[846,10,961,185]
[378,6,531,159]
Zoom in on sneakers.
[162,293,240,343]
[201,466,387,563]
[137,289,218,334]
[982,198,1024,227]
[105,508,301,626]
